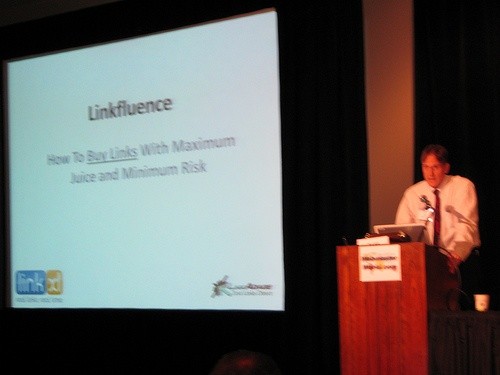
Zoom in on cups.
[474,294,489,312]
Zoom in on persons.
[210,350,281,375]
[395,145,482,310]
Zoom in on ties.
[434,193,444,246]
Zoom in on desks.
[429,310,500,375]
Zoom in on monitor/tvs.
[374,226,430,243]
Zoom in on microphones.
[420,195,433,208]
